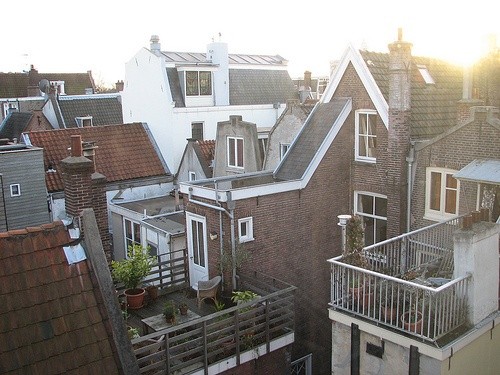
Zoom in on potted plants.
[213,240,256,291]
[344,213,455,334]
[164,303,180,328]
[211,296,234,355]
[110,241,156,309]
[122,310,132,339]
[231,290,259,327]
[144,282,158,300]
[127,327,140,350]
[179,302,190,315]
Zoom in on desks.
[141,308,202,354]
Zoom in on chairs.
[197,276,222,313]
